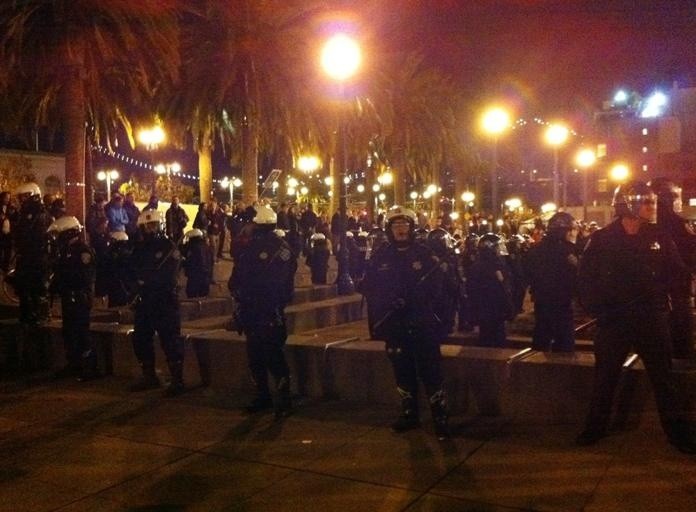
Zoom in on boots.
[391,386,420,432]
[270,367,291,415]
[247,364,272,414]
[129,353,159,393]
[427,384,452,441]
[162,356,184,396]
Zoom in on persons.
[1,177,696,450]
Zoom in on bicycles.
[1,267,18,305]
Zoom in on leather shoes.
[575,427,607,445]
[667,433,695,453]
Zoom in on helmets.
[46,216,80,232]
[414,228,535,250]
[309,230,369,240]
[13,182,41,197]
[382,204,419,229]
[136,210,161,229]
[646,177,682,199]
[612,180,655,206]
[185,228,203,238]
[546,211,577,231]
[245,205,277,224]
